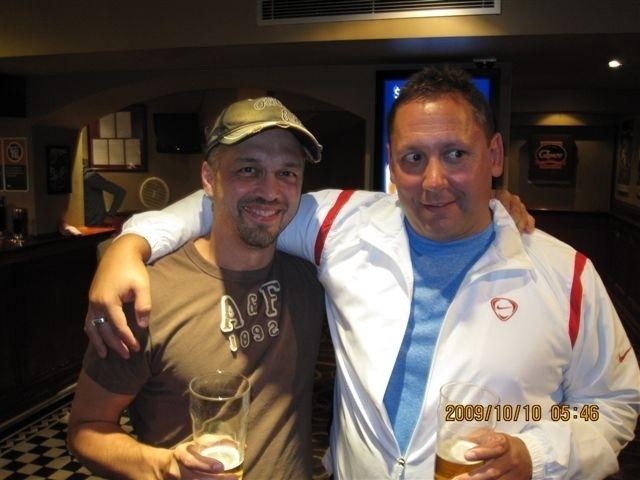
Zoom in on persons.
[65,97,535,480]
[84,65,640,480]
[83,158,126,227]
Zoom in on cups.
[188,371,251,480]
[434,381,500,480]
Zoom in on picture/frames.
[44,144,73,195]
[88,106,147,173]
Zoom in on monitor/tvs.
[153,112,203,154]
[375,70,499,197]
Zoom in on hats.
[201,95,323,166]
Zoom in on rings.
[92,317,106,327]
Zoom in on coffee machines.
[0,122,88,239]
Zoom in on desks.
[0,225,117,425]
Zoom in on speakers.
[0,73,25,117]
